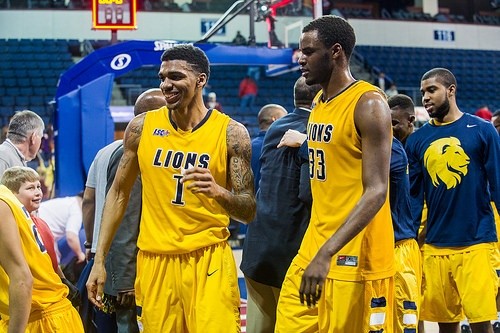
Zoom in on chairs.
[0,38,500,169]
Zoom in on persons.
[32,189,86,299]
[0,165,59,281]
[0,110,45,177]
[82,15,500,333]
[0,184,85,333]
